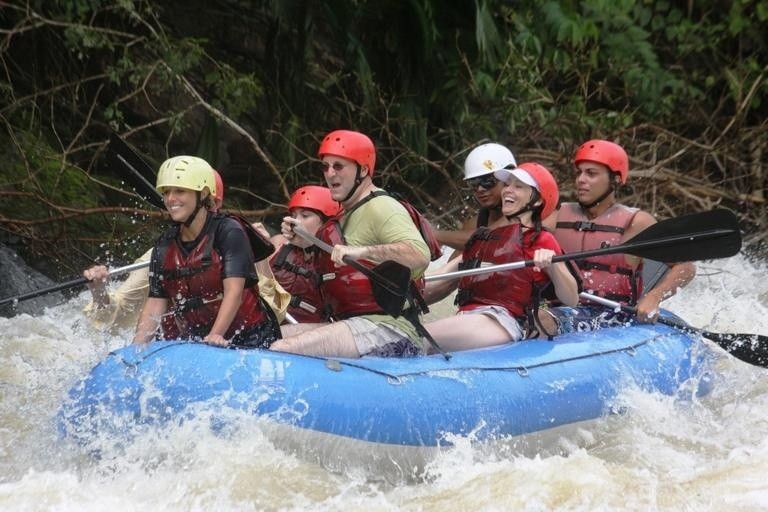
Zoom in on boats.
[54,307,717,485]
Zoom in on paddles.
[290,224,408,320]
[423,209,742,282]
[580,291,768,368]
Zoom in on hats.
[493,168,539,192]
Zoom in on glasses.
[468,175,497,192]
[319,162,354,171]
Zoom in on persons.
[537,139,696,336]
[131,155,274,350]
[82,166,291,336]
[251,185,346,325]
[422,162,584,356]
[433,142,518,264]
[267,130,431,360]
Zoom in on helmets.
[209,169,224,211]
[494,162,559,220]
[156,155,216,202]
[317,130,376,176]
[463,143,517,182]
[575,139,629,185]
[288,186,344,217]
